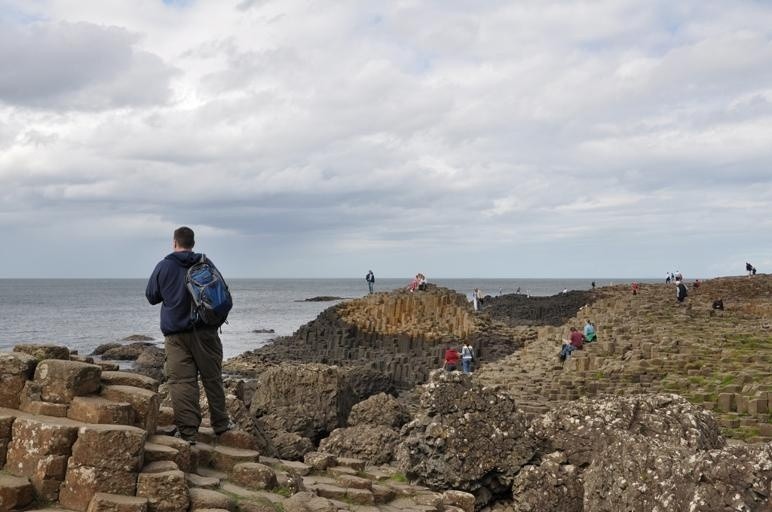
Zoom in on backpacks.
[186,252,231,327]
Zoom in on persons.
[746,263,756,278]
[562,288,567,296]
[592,280,595,288]
[559,319,597,361]
[443,341,474,372]
[365,269,375,294]
[413,274,427,291]
[631,282,638,296]
[145,225,237,447]
[473,288,483,306]
[666,270,700,301]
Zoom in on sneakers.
[215,420,234,434]
[174,430,199,445]
